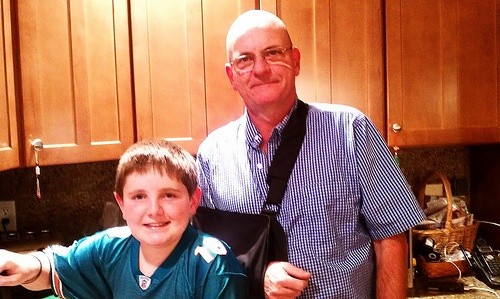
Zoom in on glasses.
[230,45,294,73]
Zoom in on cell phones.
[425,281,464,293]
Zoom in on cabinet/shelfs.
[0,0,500,174]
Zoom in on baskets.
[412,170,481,281]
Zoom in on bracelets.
[20,255,43,284]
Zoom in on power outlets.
[0,200,16,232]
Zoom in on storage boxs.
[418,255,469,278]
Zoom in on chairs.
[192,205,270,299]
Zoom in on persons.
[195,10,425,299]
[0,138,245,299]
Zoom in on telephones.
[459,239,500,288]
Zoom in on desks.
[407,261,500,299]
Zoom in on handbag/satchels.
[192,205,287,299]
